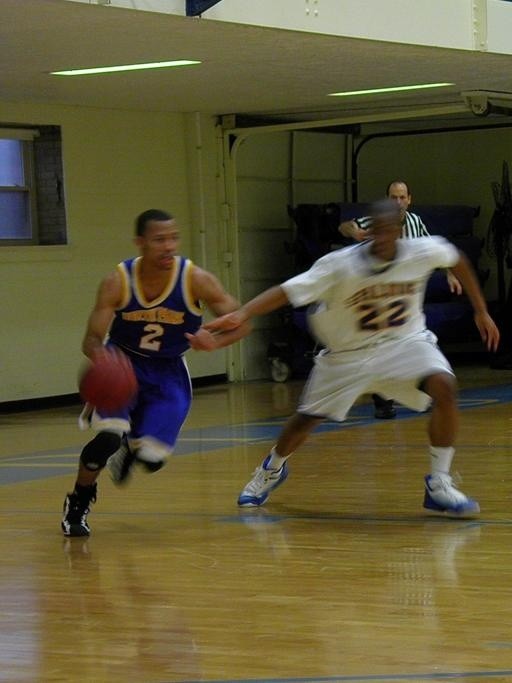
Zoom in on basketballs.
[80,356,137,411]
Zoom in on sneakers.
[423,476,479,516]
[107,436,135,485]
[237,456,288,506]
[375,407,397,420]
[60,492,92,536]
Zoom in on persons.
[194,197,500,517]
[59,208,254,539]
[336,179,464,420]
